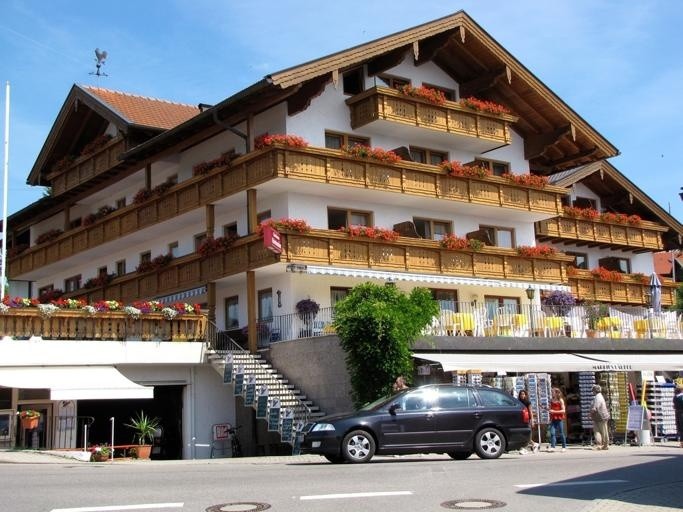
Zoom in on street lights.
[644,292,653,339]
[526,284,535,337]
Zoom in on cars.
[296,383,530,458]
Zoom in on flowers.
[563,207,641,225]
[50,156,74,173]
[339,142,401,163]
[438,232,469,250]
[1,296,203,320]
[397,85,449,106]
[296,299,320,319]
[133,182,178,205]
[440,159,491,181]
[6,243,29,257]
[37,289,66,304]
[515,245,557,259]
[33,228,66,246]
[338,224,399,241]
[255,132,309,151]
[592,266,624,284]
[90,445,110,455]
[460,96,511,119]
[81,273,119,289]
[137,253,176,275]
[82,205,118,225]
[79,134,111,157]
[15,407,40,419]
[192,145,242,176]
[196,233,239,257]
[545,289,576,316]
[502,172,550,190]
[255,218,309,234]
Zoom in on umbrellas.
[650,272,662,313]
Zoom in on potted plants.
[123,409,158,459]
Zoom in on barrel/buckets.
[640,429,650,445]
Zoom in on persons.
[539,387,566,453]
[517,390,539,455]
[591,385,610,450]
[673,384,683,448]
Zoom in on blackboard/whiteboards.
[626,406,644,431]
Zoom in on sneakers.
[519,443,611,455]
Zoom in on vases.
[22,417,39,429]
[93,454,109,462]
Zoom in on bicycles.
[225,425,242,457]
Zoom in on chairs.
[267,328,281,342]
